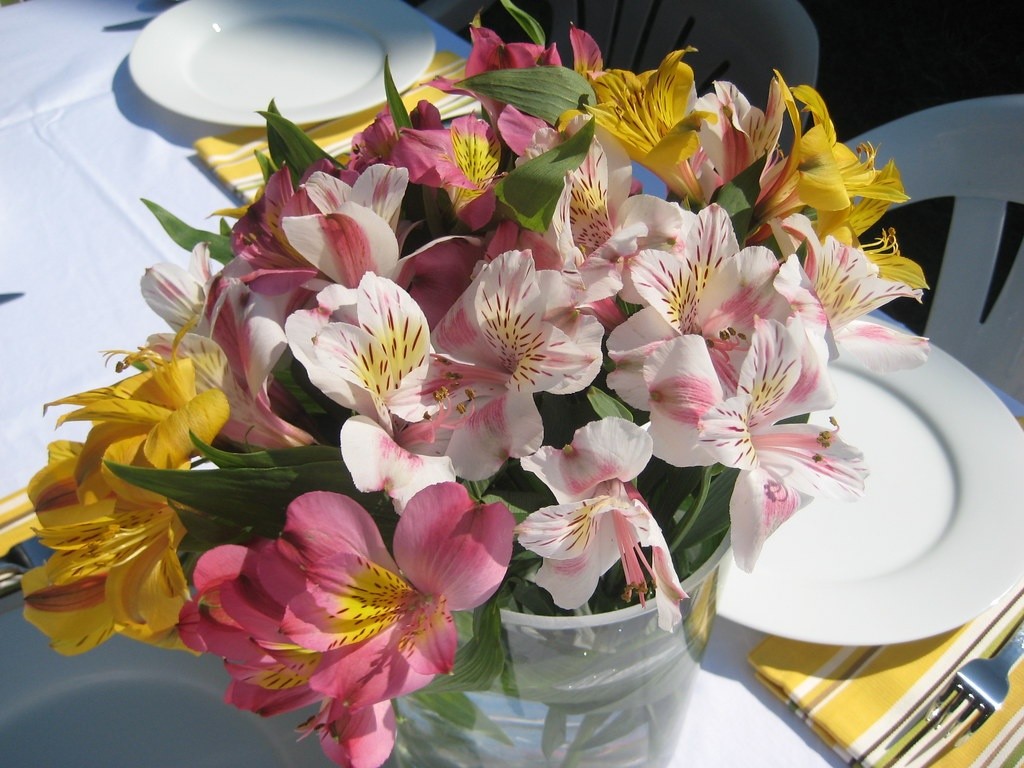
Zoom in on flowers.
[16,3,933,768]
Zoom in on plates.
[715,319,1024,648]
[128,1,436,130]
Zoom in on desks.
[0,0,1024,768]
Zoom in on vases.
[392,521,731,768]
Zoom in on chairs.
[487,2,820,161]
[825,96,1024,407]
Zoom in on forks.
[925,623,1024,748]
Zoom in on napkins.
[746,576,1024,768]
